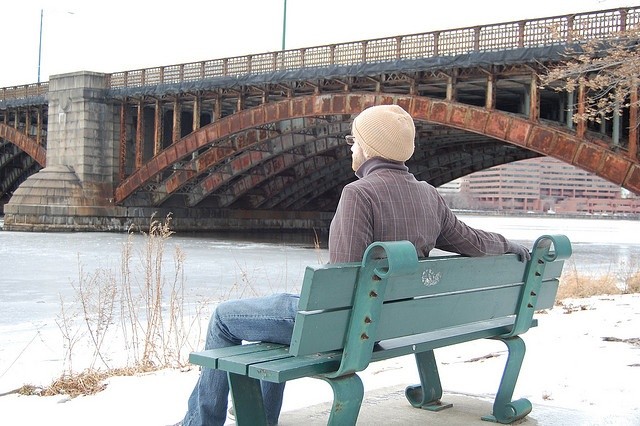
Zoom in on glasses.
[344,134,355,145]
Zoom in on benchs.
[188,234,572,426]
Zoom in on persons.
[172,105,532,426]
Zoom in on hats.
[352,105,416,161]
